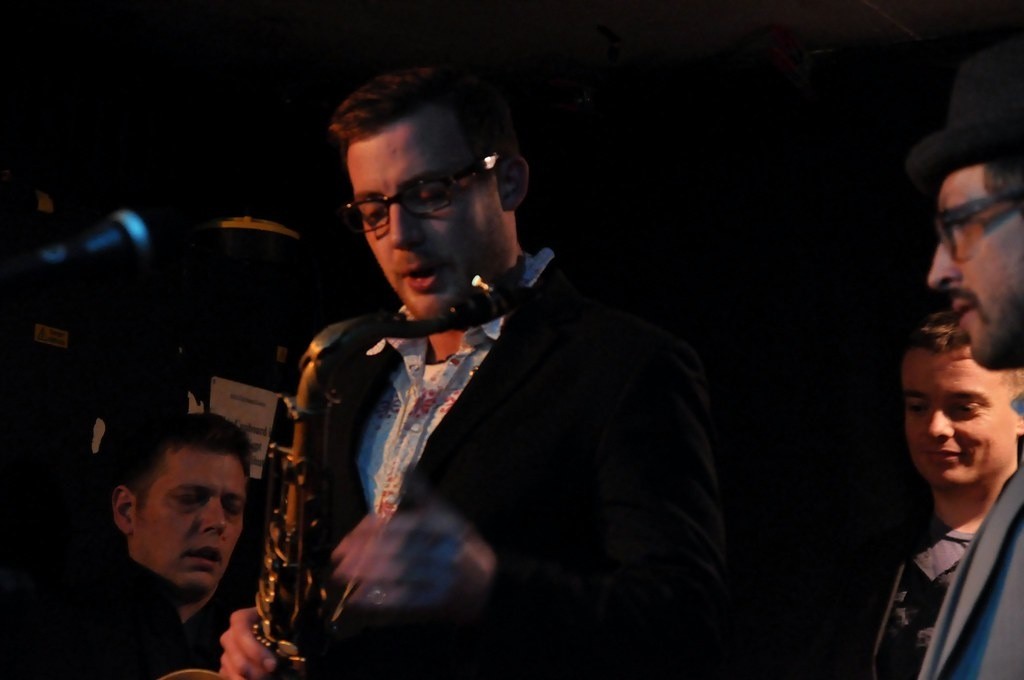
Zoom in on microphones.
[0,209,156,291]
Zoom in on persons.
[905,34,1024,680]
[217,61,726,680]
[794,310,1024,680]
[0,414,252,680]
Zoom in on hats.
[906,37,1024,197]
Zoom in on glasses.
[933,186,1024,257]
[335,151,509,233]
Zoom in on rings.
[366,586,387,606]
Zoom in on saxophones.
[157,273,544,680]
